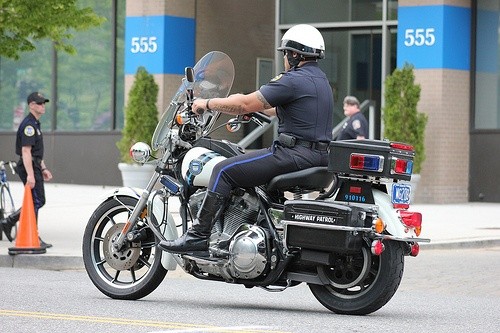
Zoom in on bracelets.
[42,168,47,172]
[206,100,211,109]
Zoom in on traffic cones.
[8,181,47,255]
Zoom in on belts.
[295,138,329,151]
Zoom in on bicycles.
[0,160,17,242]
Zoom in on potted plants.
[382,61,429,204]
[116,67,160,190]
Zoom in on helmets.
[277,24,325,59]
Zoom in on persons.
[333,96,369,139]
[0,92,52,248]
[158,23,333,256]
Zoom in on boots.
[39,236,53,248]
[0,206,21,242]
[157,187,231,257]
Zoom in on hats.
[27,91,50,103]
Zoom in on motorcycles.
[82,51,431,316]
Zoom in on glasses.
[283,49,290,55]
[32,102,45,104]
